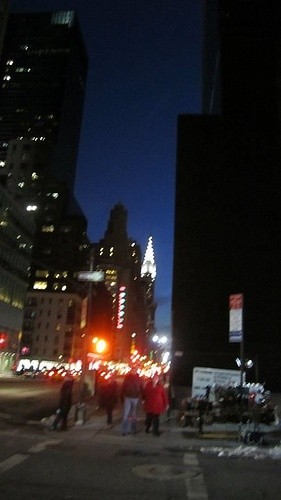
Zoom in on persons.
[118,367,145,436]
[97,376,119,429]
[142,374,169,438]
[48,375,75,433]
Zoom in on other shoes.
[51,425,56,431]
[144,428,149,434]
[132,431,137,435]
[122,432,126,436]
[105,423,112,427]
[61,424,67,430]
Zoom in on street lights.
[150,333,168,364]
